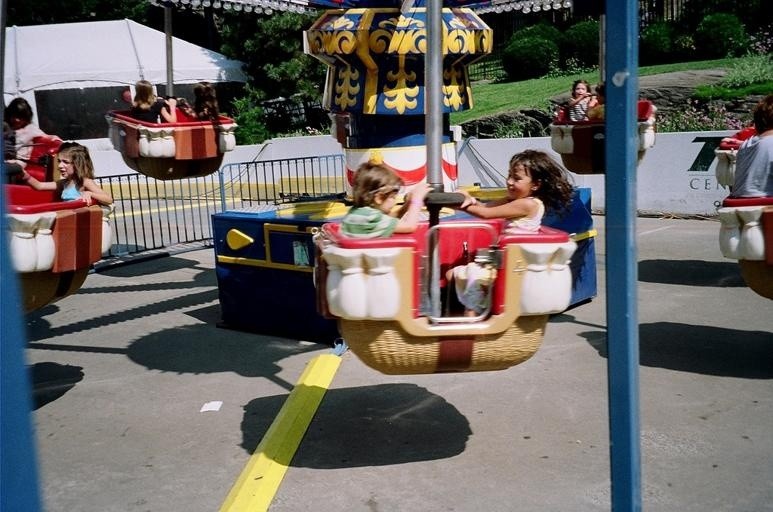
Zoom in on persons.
[445,150,573,317]
[3,98,64,168]
[22,142,112,207]
[342,164,435,240]
[721,137,744,144]
[568,80,605,121]
[735,96,773,198]
[134,81,218,123]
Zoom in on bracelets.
[734,138,736,143]
[472,197,477,205]
